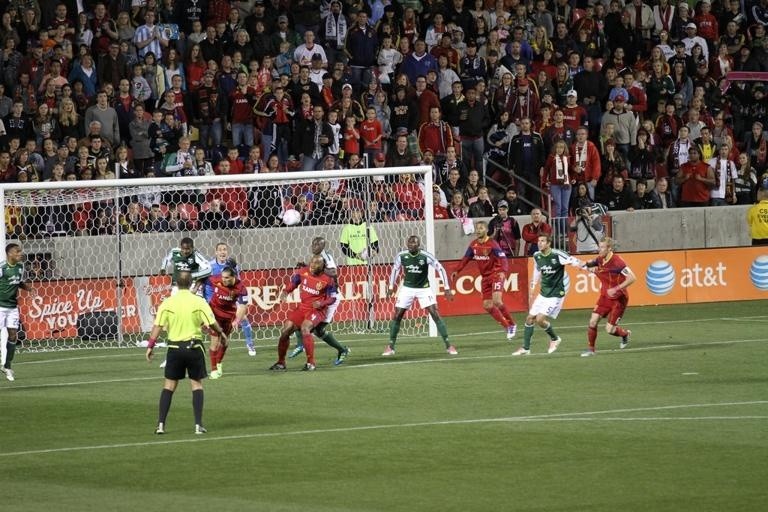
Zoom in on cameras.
[495,215,503,227]
[577,209,583,215]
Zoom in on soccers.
[284,210,301,225]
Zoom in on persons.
[201,242,256,356]
[159,237,213,370]
[191,258,248,379]
[512,233,596,355]
[450,221,518,340]
[146,271,228,436]
[1,1,768,264]
[579,236,637,356]
[268,256,337,370]
[382,236,458,355]
[0,243,32,380]
[289,237,351,366]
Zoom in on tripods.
[494,227,514,257]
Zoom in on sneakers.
[512,347,531,356]
[269,345,315,371]
[382,345,396,356]
[1,366,15,382]
[336,346,351,365]
[159,361,167,368]
[195,424,208,435]
[446,346,458,355]
[581,351,598,357]
[210,361,223,380]
[506,324,517,341]
[154,422,165,433]
[247,342,256,357]
[620,330,631,349]
[548,336,561,354]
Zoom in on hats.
[312,53,321,61]
[567,90,577,97]
[686,23,696,28]
[519,79,528,87]
[614,95,624,102]
[498,200,509,208]
[376,153,385,162]
[343,84,353,90]
